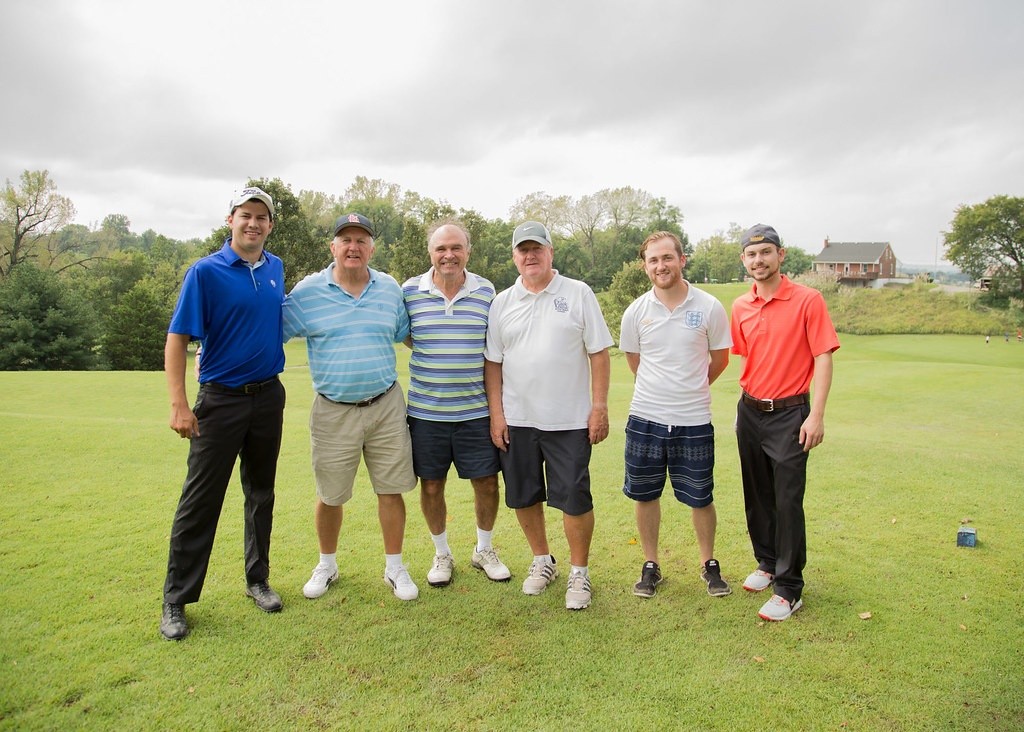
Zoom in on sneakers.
[304,563,338,598]
[472,546,511,581]
[634,561,662,597]
[428,552,454,586]
[162,601,187,640]
[523,555,558,595]
[385,564,418,600]
[759,595,802,620]
[565,569,591,610]
[246,579,282,612]
[701,560,731,596]
[743,569,775,590]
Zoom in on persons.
[619,232,735,598]
[985,324,1022,344]
[400,220,511,585]
[731,223,840,620]
[194,212,418,600]
[160,187,286,640]
[483,221,615,609]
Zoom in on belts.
[202,375,279,394]
[742,390,810,411]
[318,380,395,407]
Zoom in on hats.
[512,221,552,249]
[230,187,275,218]
[335,213,374,237]
[742,222,781,249]
